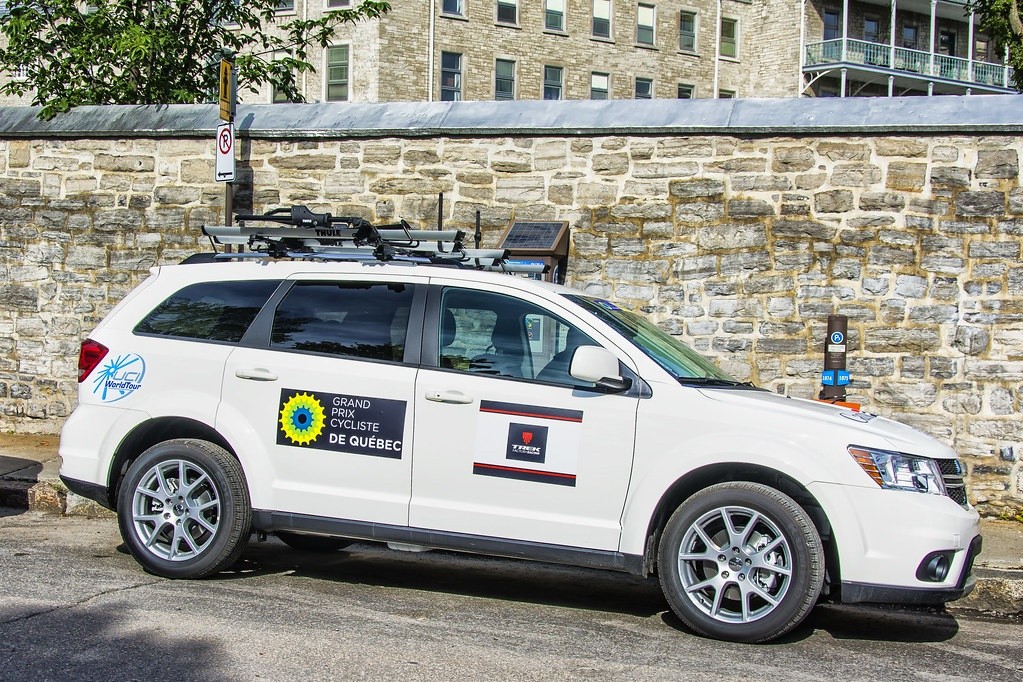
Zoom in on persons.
[535,328,600,387]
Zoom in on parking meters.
[820,314,860,412]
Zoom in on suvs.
[57,214,985,644]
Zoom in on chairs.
[469,315,525,377]
[440,310,456,369]
